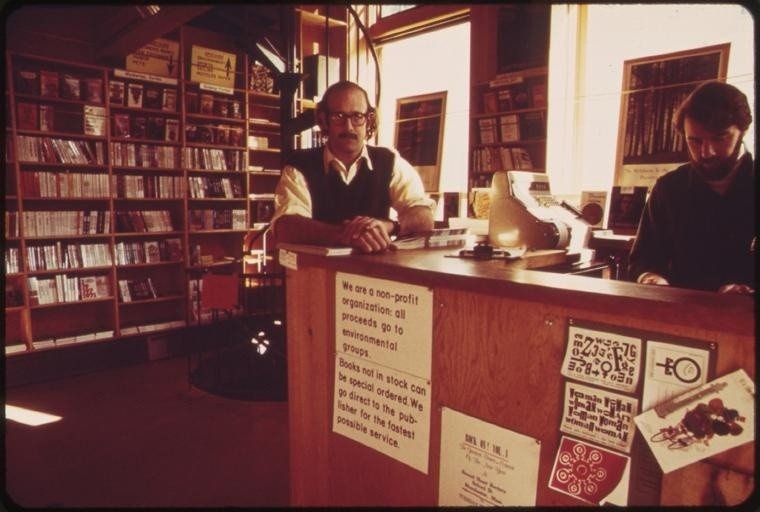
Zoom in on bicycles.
[652,422,700,450]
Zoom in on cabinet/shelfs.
[470,106,547,175]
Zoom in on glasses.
[328,112,368,126]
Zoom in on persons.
[629,80,756,294]
[269,82,434,254]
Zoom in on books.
[469,85,546,189]
[4,69,283,354]
